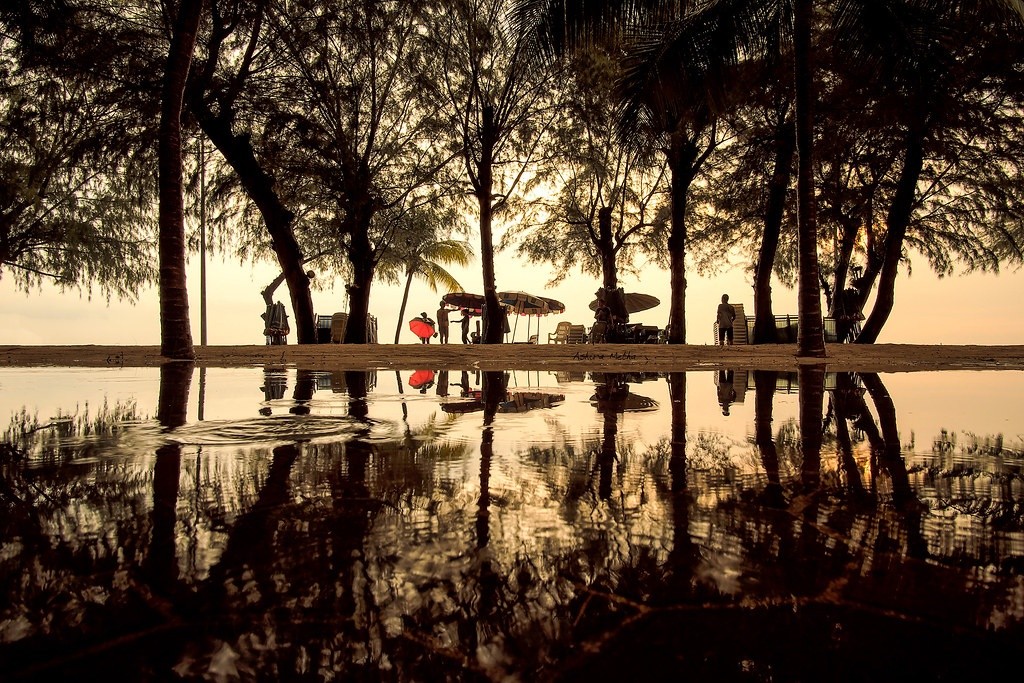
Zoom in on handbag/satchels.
[433,333,438,338]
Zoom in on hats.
[440,301,446,305]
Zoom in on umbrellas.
[259,368,288,401]
[438,369,565,414]
[588,292,660,314]
[499,290,548,343]
[533,296,565,344]
[263,301,290,345]
[410,317,434,338]
[443,293,514,344]
[408,369,435,388]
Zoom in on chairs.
[548,370,586,383]
[471,321,480,339]
[548,322,585,345]
[714,304,747,346]
[331,313,347,343]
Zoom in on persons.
[716,370,737,416]
[449,309,469,345]
[450,371,469,397]
[415,381,435,394]
[437,301,461,344]
[419,312,435,344]
[716,294,736,345]
[436,370,448,397]
[594,299,614,344]
